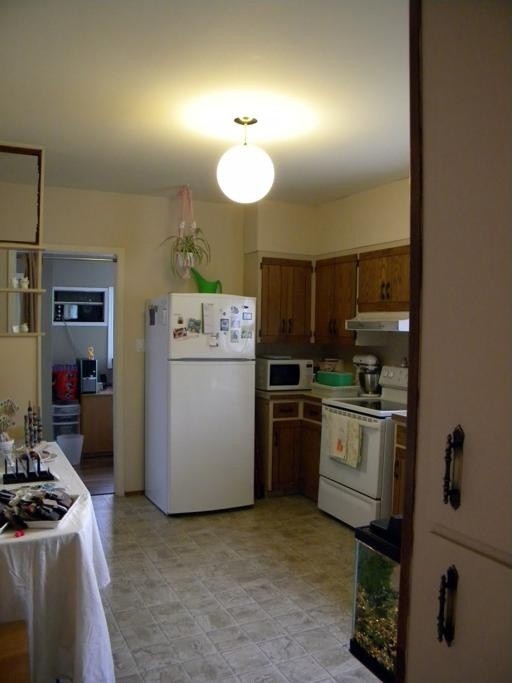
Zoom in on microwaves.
[255,357,314,391]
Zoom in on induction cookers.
[321,397,408,416]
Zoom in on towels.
[328,419,366,468]
[325,410,349,468]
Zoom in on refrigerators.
[143,292,258,520]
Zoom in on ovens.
[318,404,391,526]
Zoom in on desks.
[0,439,117,682]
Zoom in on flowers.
[0,397,22,441]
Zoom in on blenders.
[352,354,381,397]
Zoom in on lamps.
[214,114,275,206]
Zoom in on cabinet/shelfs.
[52,402,81,437]
[258,255,313,344]
[256,395,301,498]
[0,241,47,443]
[79,395,113,458]
[314,252,358,347]
[405,0,512,571]
[301,395,321,502]
[358,242,410,313]
[396,517,512,682]
[390,420,407,518]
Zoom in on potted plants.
[158,225,213,280]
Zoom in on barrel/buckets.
[58,433,83,468]
[52,360,77,404]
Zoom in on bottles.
[401,356,407,367]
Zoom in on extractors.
[344,310,410,331]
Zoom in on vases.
[0,439,15,453]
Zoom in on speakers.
[77,359,97,393]
